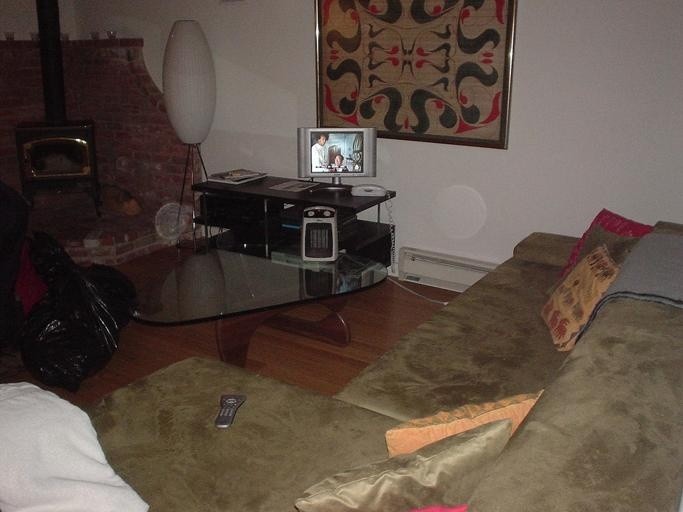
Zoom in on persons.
[328,154,348,173]
[309,133,331,168]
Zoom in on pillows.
[295,418,513,512]
[558,210,654,283]
[385,389,543,458]
[541,240,620,353]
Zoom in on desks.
[191,174,396,271]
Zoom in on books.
[218,169,260,179]
[203,167,268,186]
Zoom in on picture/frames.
[313,0,519,149]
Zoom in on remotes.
[215,395,245,428]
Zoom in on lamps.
[163,19,221,257]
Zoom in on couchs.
[0,221,683,512]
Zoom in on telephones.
[352,184,386,197]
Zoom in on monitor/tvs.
[297,127,377,190]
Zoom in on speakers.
[300,205,339,262]
